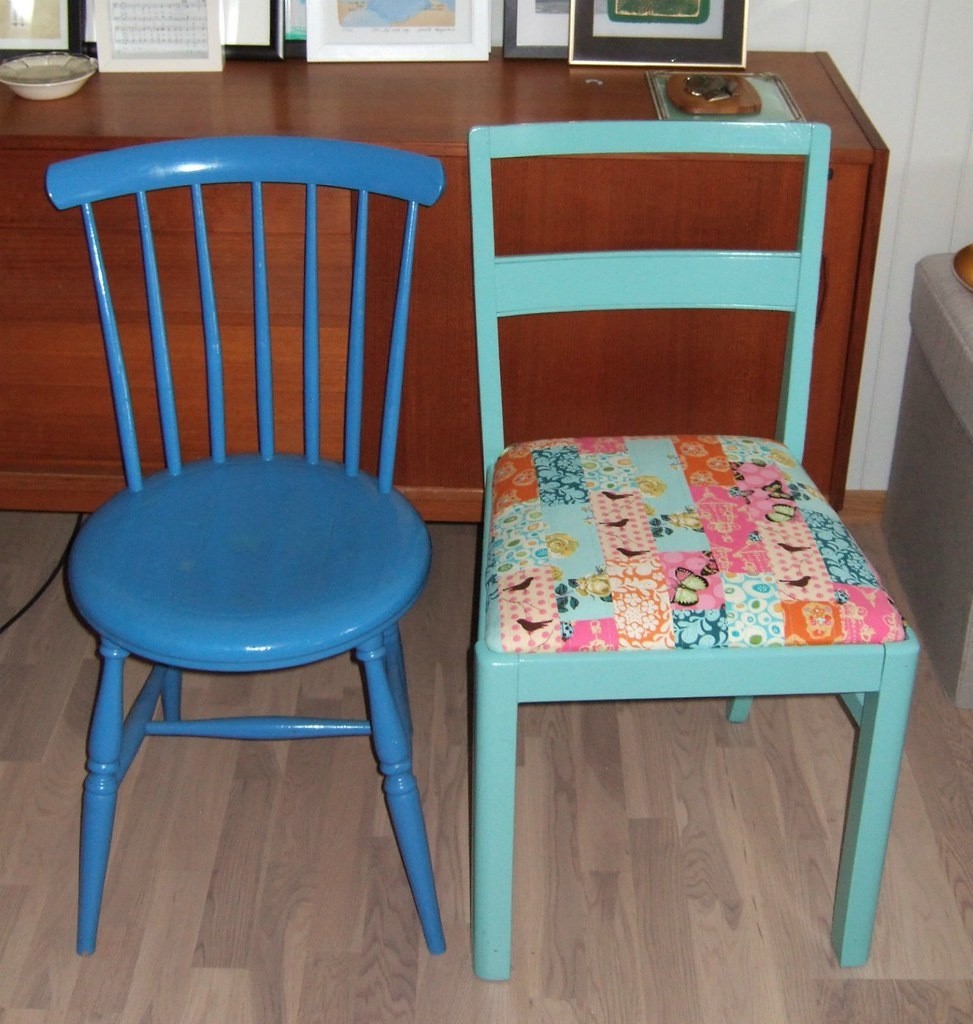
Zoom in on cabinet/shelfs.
[0,43,889,523]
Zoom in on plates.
[951,240,973,296]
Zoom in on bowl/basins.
[0,51,97,100]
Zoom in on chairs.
[467,120,919,982]
[45,139,447,957]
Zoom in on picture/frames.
[0,0,749,74]
